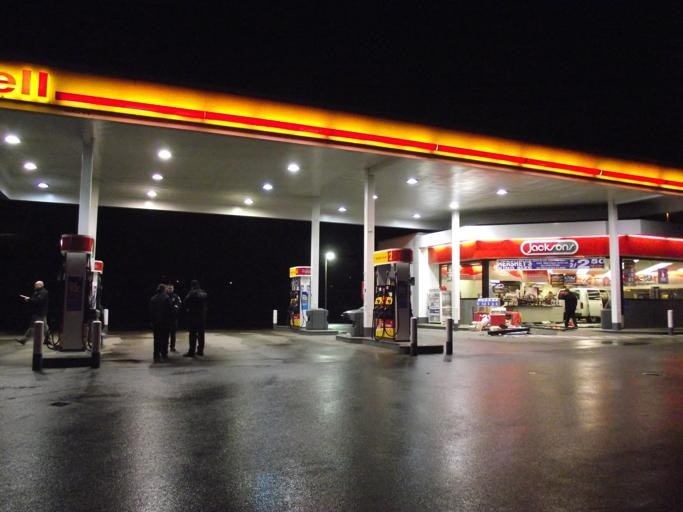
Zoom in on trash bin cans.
[342,310,363,337]
[600,310,611,329]
[305,308,327,329]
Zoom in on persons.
[182,280,208,357]
[150,284,173,361]
[166,284,182,351]
[16,281,52,345]
[558,289,577,331]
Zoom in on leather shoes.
[153,347,203,361]
[15,336,25,345]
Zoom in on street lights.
[325,252,335,309]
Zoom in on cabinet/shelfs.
[427,290,451,325]
